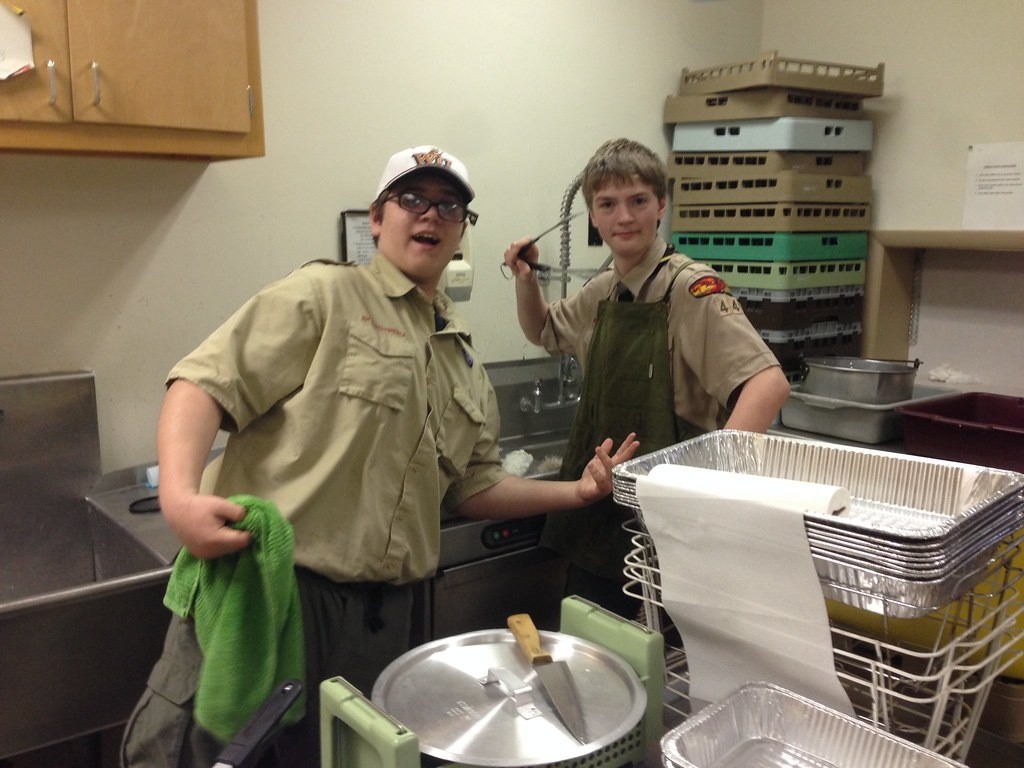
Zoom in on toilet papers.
[633,462,861,725]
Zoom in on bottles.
[447,252,471,303]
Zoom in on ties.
[616,281,635,303]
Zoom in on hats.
[375,145,475,204]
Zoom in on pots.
[796,353,919,404]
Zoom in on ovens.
[419,513,551,642]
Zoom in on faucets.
[520,352,586,413]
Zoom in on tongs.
[520,210,612,278]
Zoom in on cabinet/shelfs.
[0,0,265,161]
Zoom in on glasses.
[381,192,478,226]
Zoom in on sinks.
[495,427,576,480]
[894,392,1023,474]
[781,377,963,445]
[0,497,170,613]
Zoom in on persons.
[503,139,792,630]
[119,146,639,768]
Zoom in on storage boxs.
[781,379,960,445]
[896,391,1024,473]
[663,49,885,373]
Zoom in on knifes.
[507,613,590,745]
